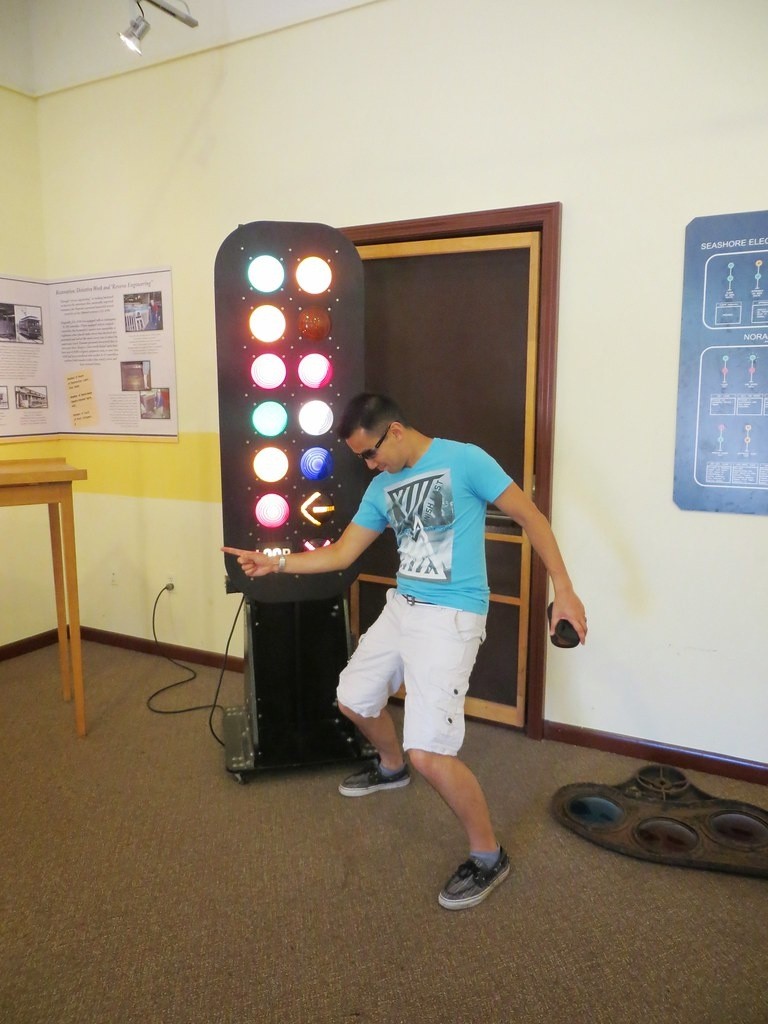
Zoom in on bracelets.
[278,555,286,575]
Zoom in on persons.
[151,300,158,326]
[153,389,163,418]
[142,362,150,390]
[221,395,587,910]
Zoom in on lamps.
[117,0,199,57]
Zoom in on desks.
[0,456,88,740]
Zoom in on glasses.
[355,425,391,460]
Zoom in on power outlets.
[164,575,177,592]
[108,568,119,585]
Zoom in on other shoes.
[339,758,411,797]
[437,841,510,911]
